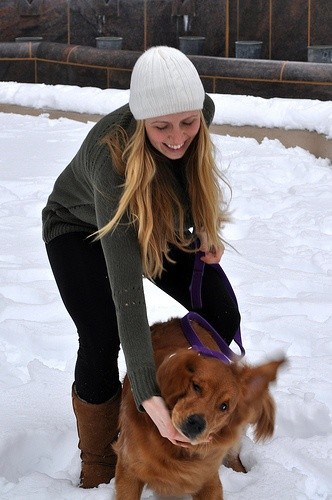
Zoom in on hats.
[128,46,205,120]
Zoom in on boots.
[222,455,248,473]
[71,381,123,489]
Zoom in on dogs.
[111,316,286,500]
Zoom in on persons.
[41,46,248,489]
